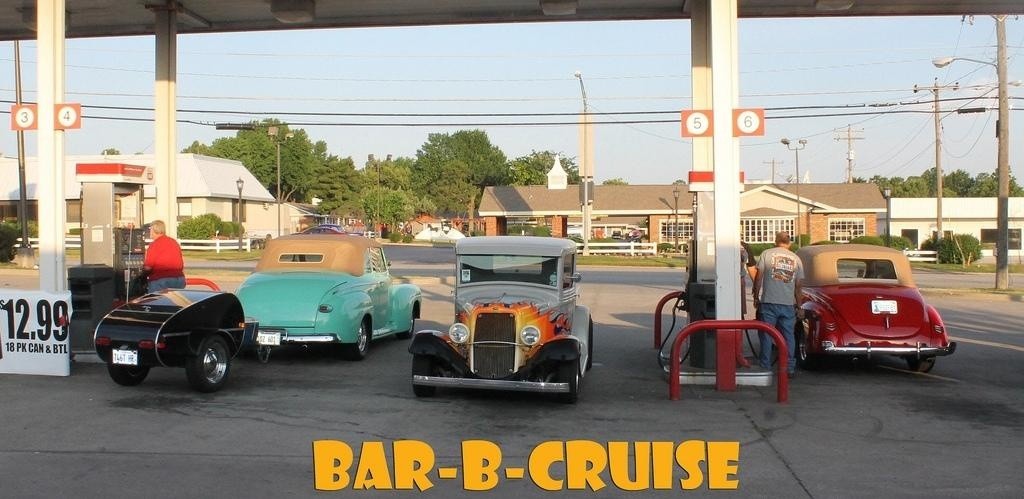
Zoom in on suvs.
[401,228,600,409]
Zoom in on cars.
[229,223,427,363]
[788,238,957,377]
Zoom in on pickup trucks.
[612,231,621,239]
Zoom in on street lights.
[933,79,1024,241]
[276,132,295,237]
[928,55,1012,291]
[673,184,681,252]
[777,138,810,249]
[574,67,589,259]
[235,175,244,255]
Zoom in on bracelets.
[754,298,759,301]
[796,305,804,310]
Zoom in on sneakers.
[787,372,795,379]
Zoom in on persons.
[740,239,759,320]
[141,220,187,294]
[753,231,806,379]
